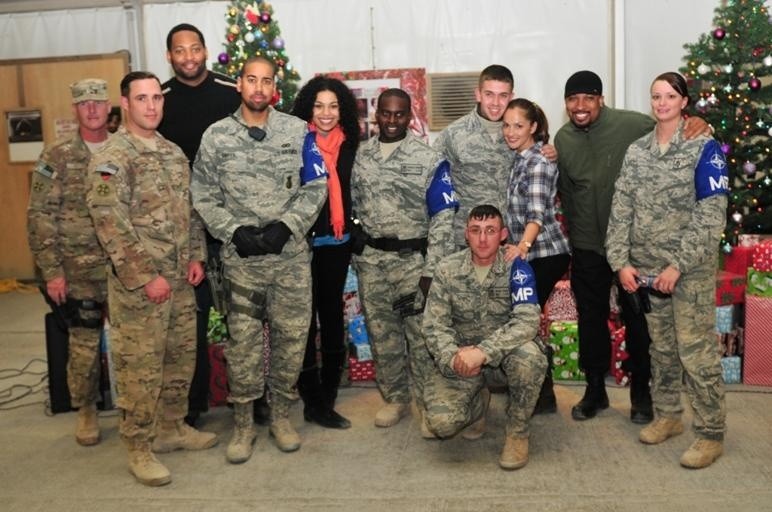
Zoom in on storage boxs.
[538,233,772,388]
[347,314,378,382]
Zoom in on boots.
[498,437,529,469]
[375,402,412,427]
[75,409,101,446]
[571,386,609,419]
[421,410,436,438]
[461,387,491,440]
[639,417,684,443]
[269,419,301,451]
[151,423,219,453]
[127,441,172,485]
[679,432,724,468]
[630,386,654,422]
[226,426,257,463]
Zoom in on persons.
[191,52,332,466]
[421,202,553,472]
[286,77,360,429]
[25,76,124,447]
[549,69,718,426]
[430,64,562,249]
[604,70,732,470]
[347,89,458,429]
[86,70,224,489]
[500,97,563,419]
[140,20,272,431]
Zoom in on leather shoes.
[304,405,351,429]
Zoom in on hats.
[71,78,109,104]
[565,70,602,98]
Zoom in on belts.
[368,237,428,252]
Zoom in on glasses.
[467,226,501,235]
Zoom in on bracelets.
[522,240,531,248]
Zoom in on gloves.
[233,225,261,258]
[258,222,293,255]
[392,276,433,318]
